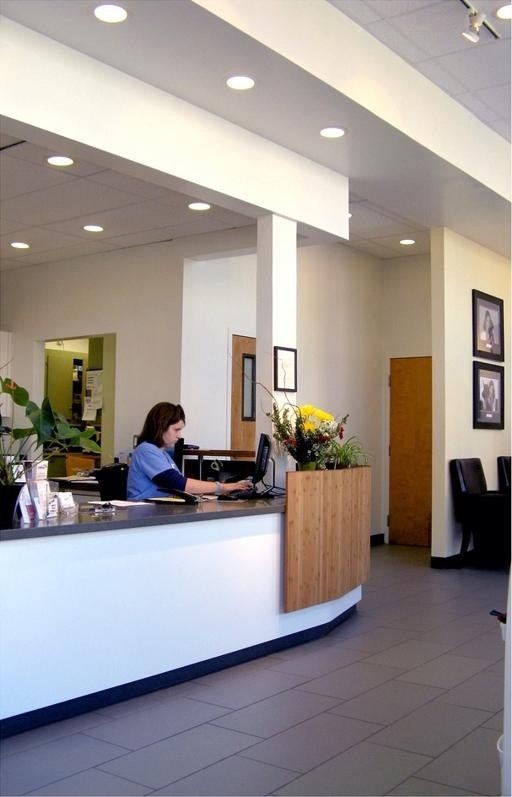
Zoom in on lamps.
[462,7,486,44]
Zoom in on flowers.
[230,352,350,464]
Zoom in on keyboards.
[229,490,250,497]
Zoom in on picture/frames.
[274,346,297,393]
[472,288,504,430]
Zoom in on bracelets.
[215,481,222,495]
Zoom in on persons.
[125,402,255,502]
[482,380,495,410]
[484,311,497,347]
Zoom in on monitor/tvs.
[242,434,275,499]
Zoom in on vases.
[296,462,317,470]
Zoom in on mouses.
[217,496,233,500]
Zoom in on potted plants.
[324,434,376,469]
[0,376,104,530]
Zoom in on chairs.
[98,463,129,501]
[448,456,511,569]
